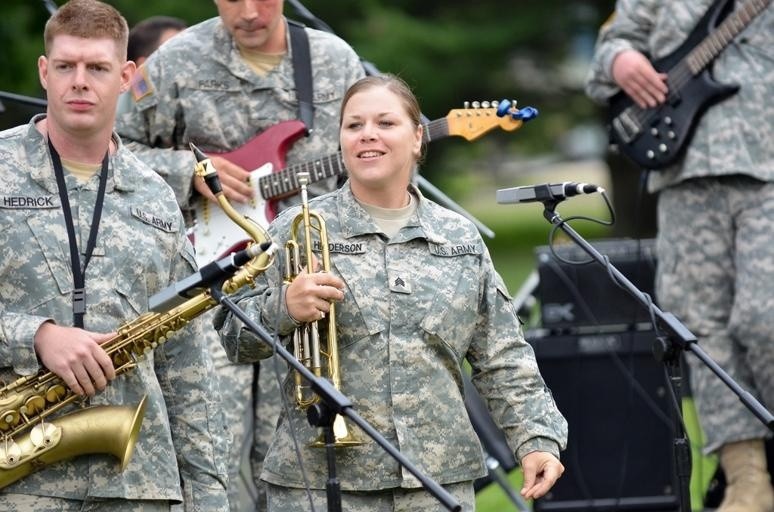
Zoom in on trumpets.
[284,172,364,448]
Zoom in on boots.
[715,439,773,512]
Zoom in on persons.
[126,13,190,69]
[213,71,571,512]
[108,0,369,512]
[1,0,236,511]
[578,0,774,512]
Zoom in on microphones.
[496,182,599,204]
[148,242,273,313]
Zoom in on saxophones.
[0,140,281,494]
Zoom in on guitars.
[180,100,539,270]
[608,0,772,168]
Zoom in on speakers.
[530,239,691,336]
[523,331,692,512]
